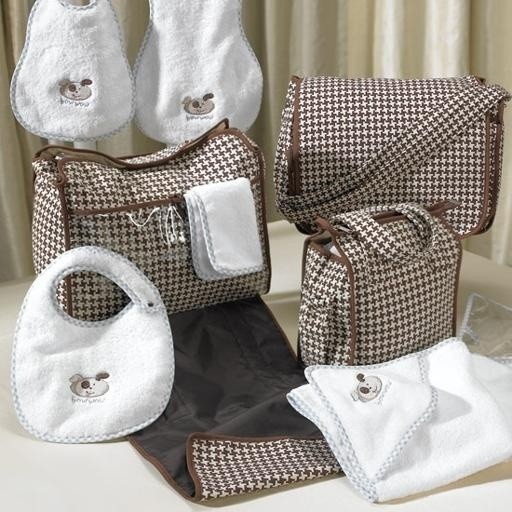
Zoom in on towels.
[285,337,512,504]
[184,177,265,282]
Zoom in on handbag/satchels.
[299,203,462,366]
[273,75,504,236]
[30,120,271,320]
